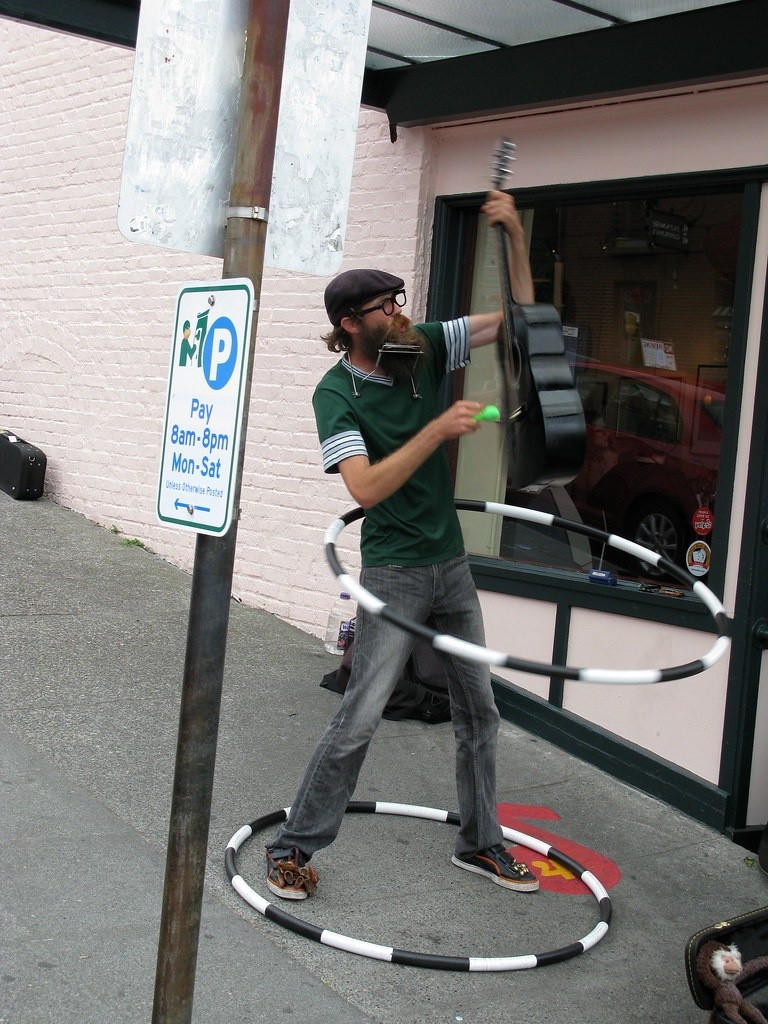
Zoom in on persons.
[264,188,540,898]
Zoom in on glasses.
[354,289,407,316]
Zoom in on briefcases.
[0,429,47,501]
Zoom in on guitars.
[487,131,587,495]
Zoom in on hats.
[324,269,405,327]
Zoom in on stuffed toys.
[697,940,768,1024]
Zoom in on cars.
[511,361,730,587]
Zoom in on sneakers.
[451,843,539,892]
[264,844,308,899]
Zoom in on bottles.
[324,593,356,655]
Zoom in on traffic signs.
[158,276,254,538]
